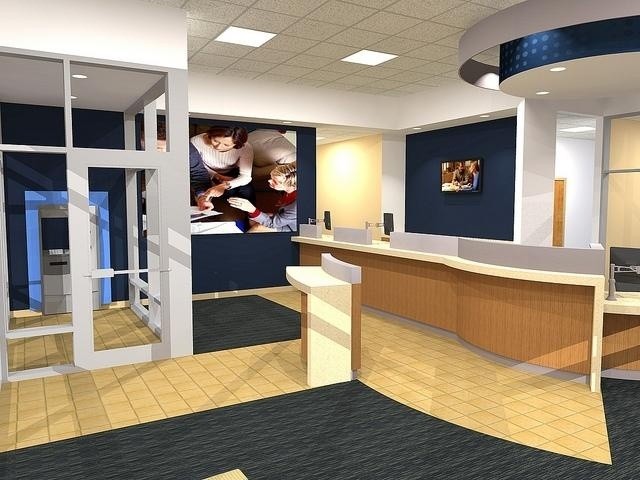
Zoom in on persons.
[141,125,216,212]
[190,124,256,233]
[451,161,469,186]
[227,164,297,233]
[456,160,480,191]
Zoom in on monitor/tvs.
[324,211,331,230]
[384,213,393,235]
[610,247,640,292]
[440,156,483,194]
